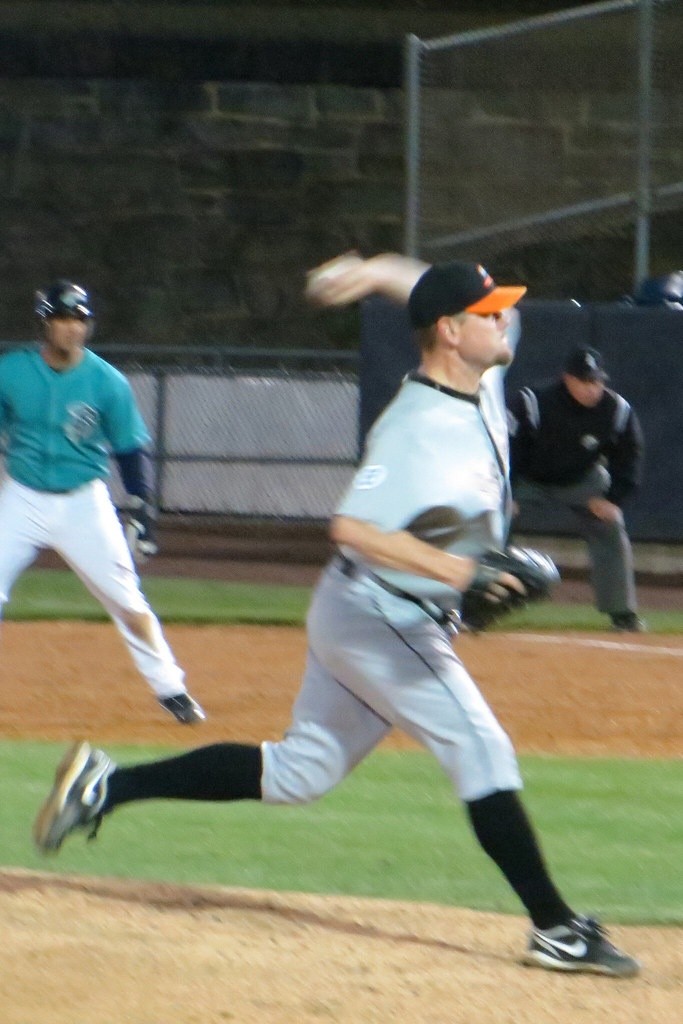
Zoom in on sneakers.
[523,913,639,978]
[159,692,207,726]
[33,737,117,854]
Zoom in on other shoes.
[610,609,648,632]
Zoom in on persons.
[502,351,651,634]
[1,276,210,733]
[32,243,646,991]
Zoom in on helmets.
[33,280,95,340]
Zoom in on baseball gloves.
[463,543,563,632]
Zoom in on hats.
[407,257,529,332]
[567,344,610,381]
